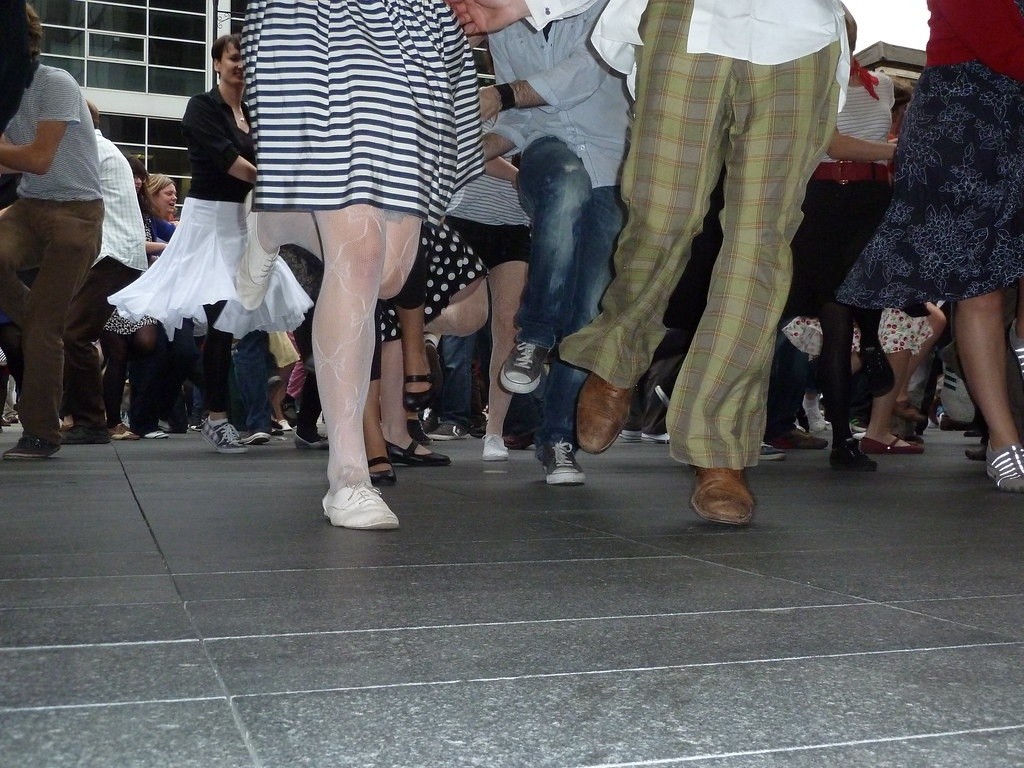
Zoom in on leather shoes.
[689,463,755,525]
[236,188,279,311]
[576,373,631,455]
[322,482,400,530]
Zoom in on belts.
[812,160,887,185]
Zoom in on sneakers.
[543,438,587,485]
[499,339,549,394]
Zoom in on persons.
[0,1,1022,532]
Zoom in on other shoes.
[0,316,1024,492]
